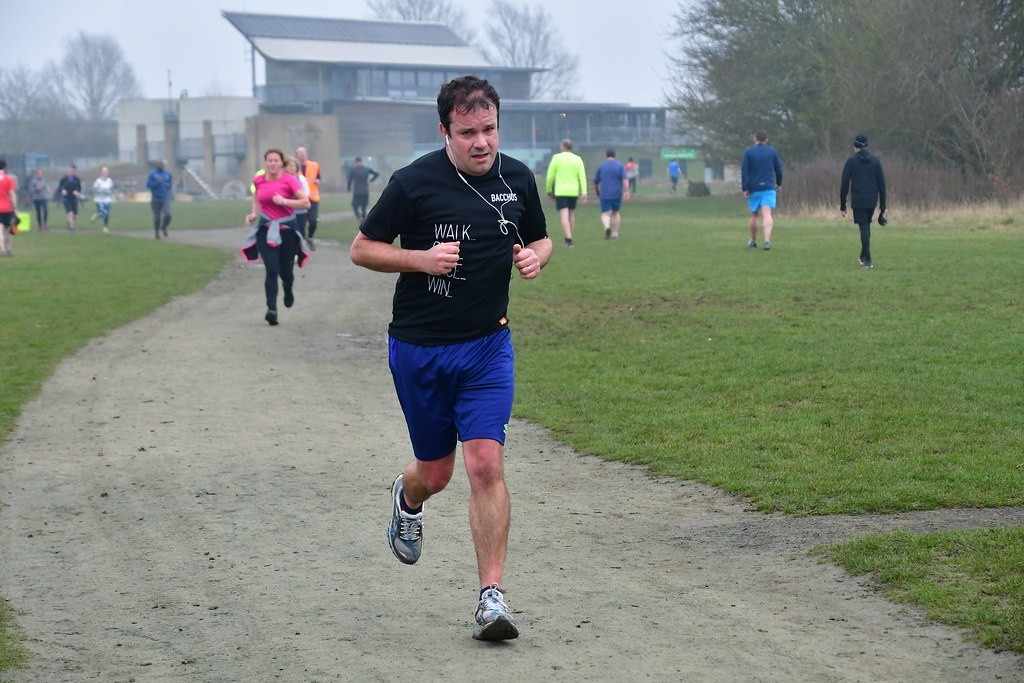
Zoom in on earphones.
[446,134,449,145]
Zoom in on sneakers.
[472,581,519,641]
[387,474,424,565]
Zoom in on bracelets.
[283,200,287,205]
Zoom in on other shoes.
[605,227,611,241]
[283,288,294,308]
[264,309,278,326]
[90,214,96,221]
[762,240,772,251]
[155,232,160,239]
[613,230,620,239]
[103,228,109,235]
[162,227,168,237]
[748,240,757,248]
[307,238,315,250]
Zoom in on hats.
[853,134,869,148]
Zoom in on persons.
[741,130,783,249]
[30,169,49,231]
[57,164,81,229]
[147,159,173,239]
[839,136,886,268]
[546,138,587,247]
[594,149,630,239]
[667,157,682,190]
[351,77,553,640]
[347,157,379,219]
[91,166,113,232]
[241,147,321,325]
[625,157,639,193]
[0,159,18,257]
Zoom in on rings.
[528,266,530,273]
